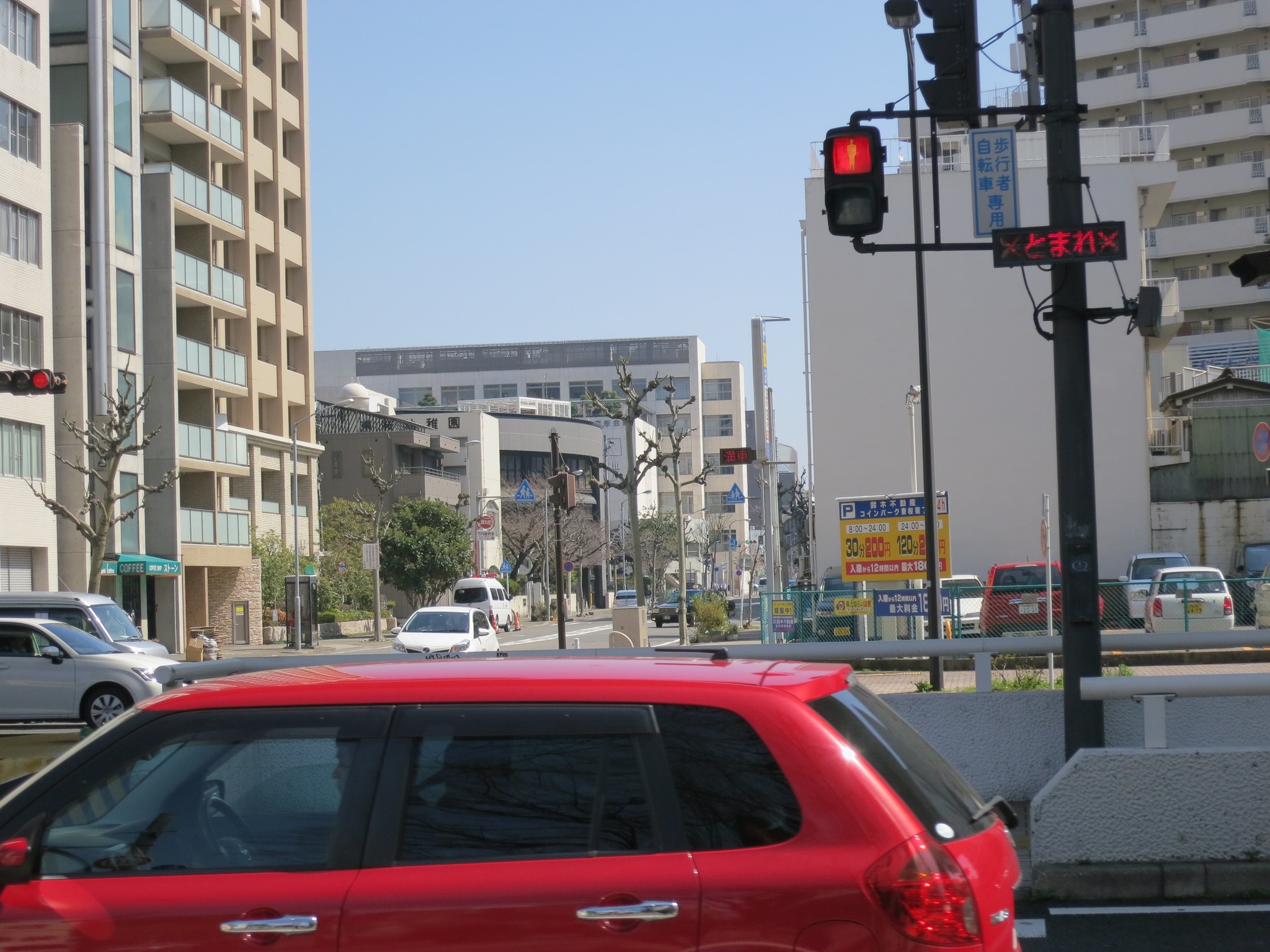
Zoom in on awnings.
[101,555,181,576]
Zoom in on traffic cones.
[516,612,520,620]
[492,617,500,634]
[512,613,521,632]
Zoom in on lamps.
[98,457,108,467]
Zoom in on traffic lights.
[823,126,884,237]
[914,0,983,129]
[0,367,55,395]
[745,539,758,544]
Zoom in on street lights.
[620,490,751,597]
[543,469,584,621]
[464,439,480,578]
[904,383,924,640]
[291,398,355,651]
[882,2,944,690]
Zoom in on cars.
[390,605,500,653]
[922,573,985,640]
[1247,563,1270,630]
[1138,566,1234,634]
[0,613,198,733]
[650,585,736,628]
[613,590,649,611]
[0,660,1025,952]
[978,560,1105,640]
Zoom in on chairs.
[1139,567,1153,576]
[1002,575,1017,585]
[1166,583,1179,594]
[833,583,846,595]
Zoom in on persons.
[721,581,728,597]
[645,585,652,607]
[751,580,757,595]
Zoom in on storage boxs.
[184,637,204,662]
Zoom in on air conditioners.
[1150,429,1168,453]
[798,555,810,578]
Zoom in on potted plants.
[531,601,557,621]
[691,593,738,642]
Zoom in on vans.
[450,573,513,633]
[1119,551,1204,629]
[759,577,767,594]
[812,566,858,643]
[0,591,171,658]
[1229,541,1270,625]
